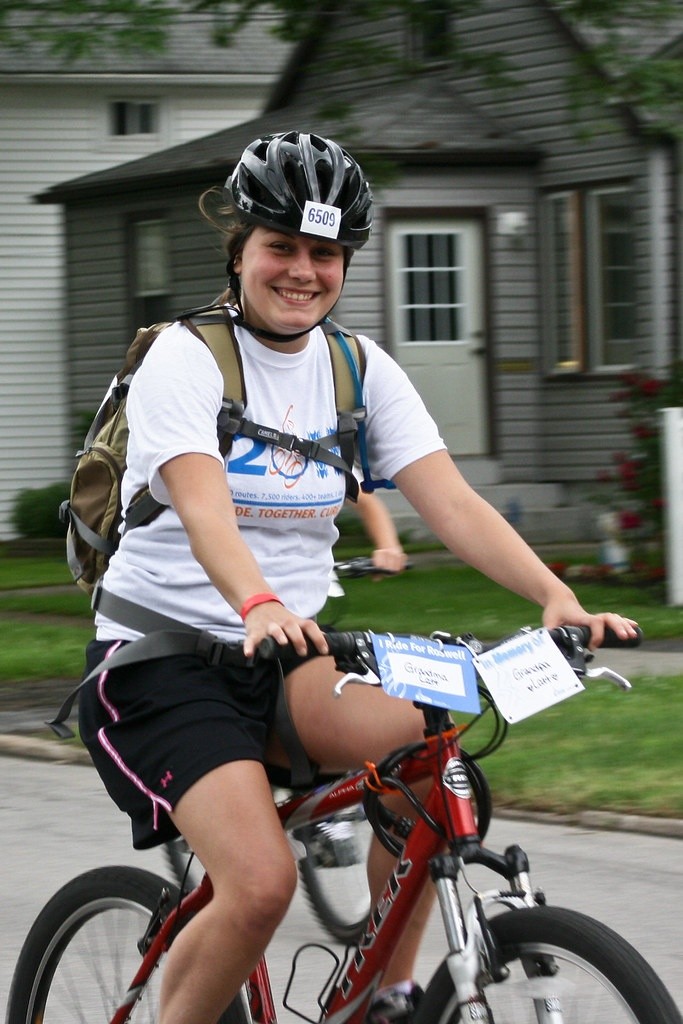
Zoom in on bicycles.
[1,620,683,1024]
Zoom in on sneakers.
[294,816,360,869]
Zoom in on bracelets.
[238,592,286,622]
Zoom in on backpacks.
[66,293,367,596]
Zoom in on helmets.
[228,130,375,250]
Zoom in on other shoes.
[366,980,423,1024]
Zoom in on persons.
[81,130,638,1023]
